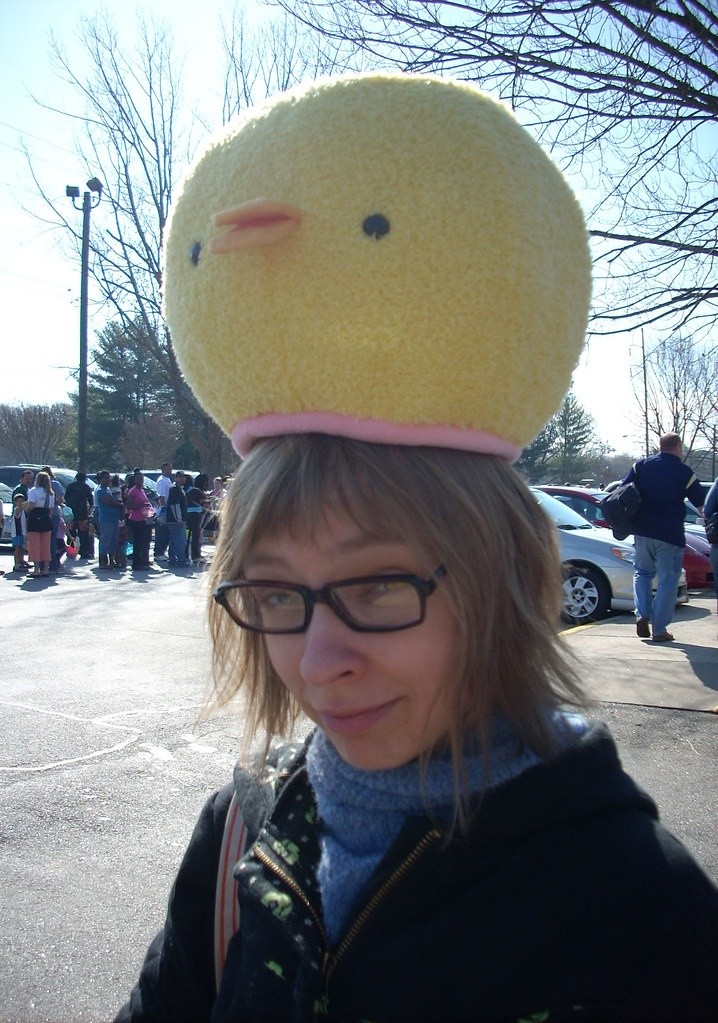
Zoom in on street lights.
[66,177,106,473]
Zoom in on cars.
[526,485,691,625]
[529,483,715,592]
[0,463,200,550]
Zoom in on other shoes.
[22,561,33,568]
[49,568,64,574]
[132,561,154,571]
[652,632,675,642]
[40,571,49,578]
[26,572,42,578]
[193,558,206,563]
[13,564,27,572]
[99,562,113,570]
[636,618,651,638]
[154,554,170,561]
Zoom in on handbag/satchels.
[153,506,167,524]
[703,511,718,544]
[64,540,79,559]
[600,471,643,534]
[26,507,53,534]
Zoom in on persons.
[565,483,570,487]
[586,484,592,488]
[702,478,718,598]
[620,432,705,642]
[0,499,5,538]
[94,463,227,568]
[111,431,718,1023]
[599,483,604,491]
[12,467,94,578]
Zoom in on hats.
[175,471,190,478]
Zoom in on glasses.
[211,562,448,635]
